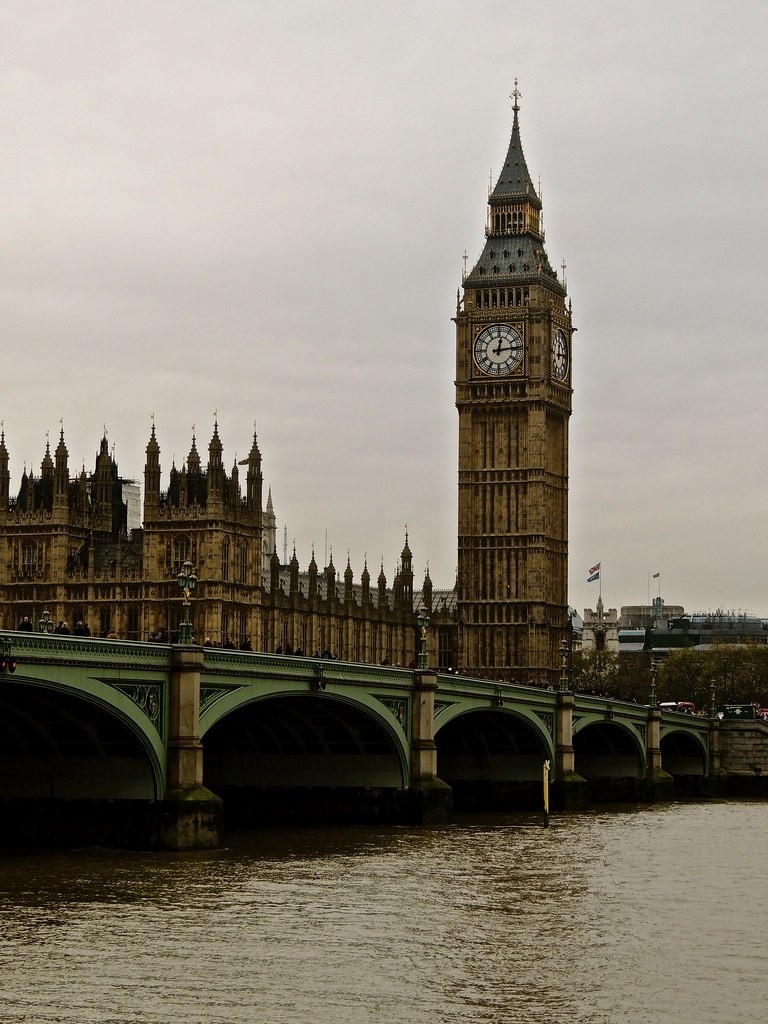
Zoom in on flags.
[653,572,659,578]
[587,562,600,583]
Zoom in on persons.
[107,628,119,639]
[665,706,673,712]
[591,689,611,698]
[382,655,389,666]
[204,636,254,652]
[684,706,709,717]
[73,621,91,636]
[275,643,303,656]
[147,626,181,644]
[313,647,340,660]
[54,621,70,635]
[409,659,417,668]
[18,616,33,631]
[509,677,552,690]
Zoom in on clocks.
[474,323,524,376]
[553,330,568,380]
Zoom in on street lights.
[559,639,569,691]
[710,676,717,719]
[417,607,430,670]
[648,663,658,707]
[177,557,197,644]
